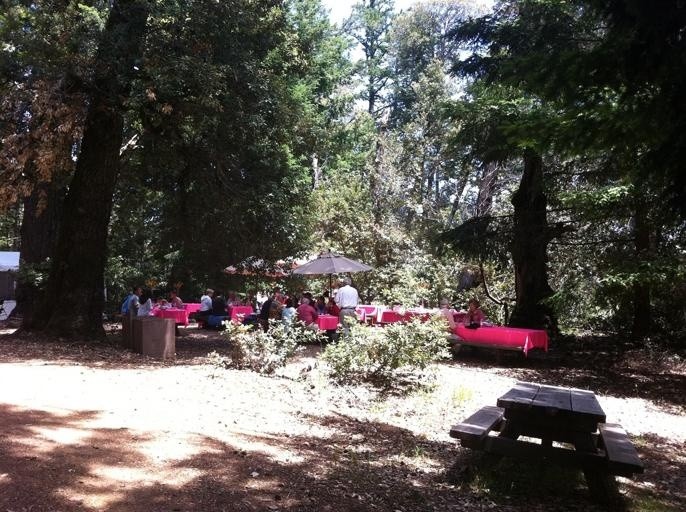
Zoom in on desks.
[447,380,630,512]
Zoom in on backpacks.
[121,295,132,316]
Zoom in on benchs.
[448,404,515,460]
[566,420,648,476]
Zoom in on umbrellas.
[293,247,375,304]
[220,253,301,280]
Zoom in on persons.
[119,285,339,339]
[333,277,360,339]
[461,299,486,362]
[437,298,463,361]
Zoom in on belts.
[341,307,357,310]
